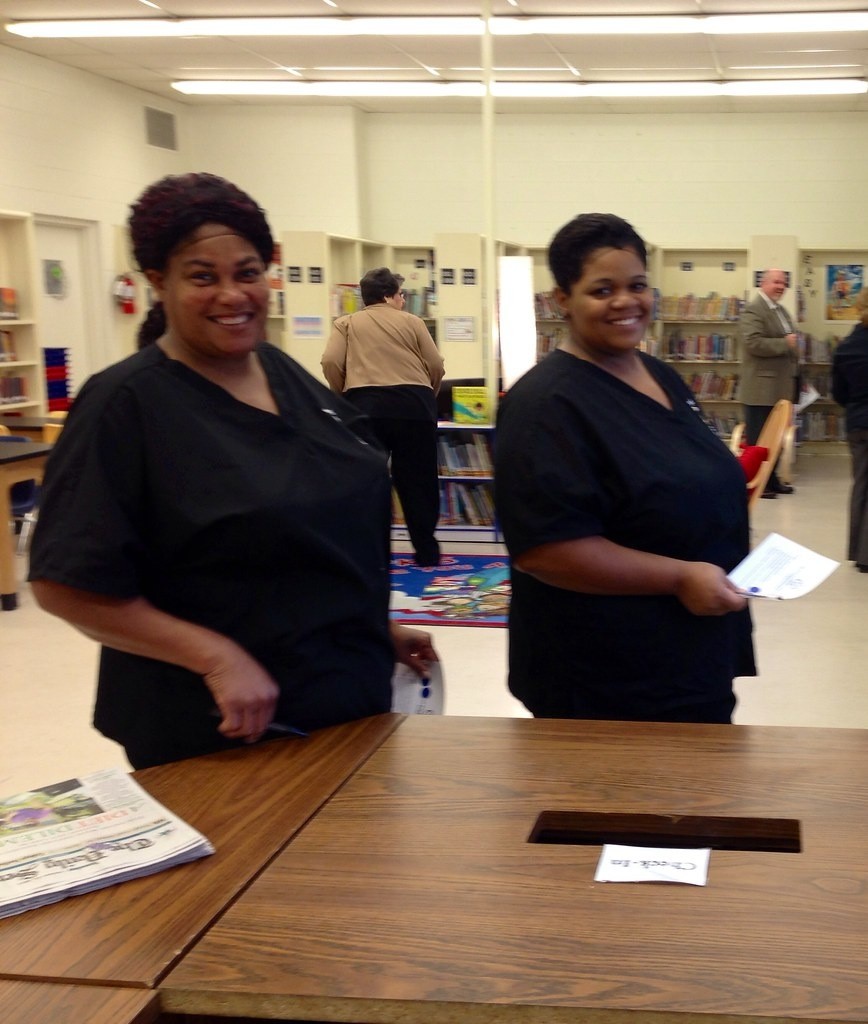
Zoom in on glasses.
[394,291,405,298]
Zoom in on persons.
[321,268,445,564]
[740,268,809,500]
[27,174,437,771]
[491,213,754,725]
[828,286,868,571]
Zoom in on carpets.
[387,551,510,627]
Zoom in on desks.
[0,713,867,1024]
[0,441,57,610]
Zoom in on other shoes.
[765,481,793,493]
[413,546,440,567]
[761,488,776,498]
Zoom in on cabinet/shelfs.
[0,211,48,416]
[383,421,506,544]
[258,232,853,456]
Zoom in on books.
[800,331,833,363]
[437,435,492,525]
[534,292,744,436]
[0,376,25,397]
[811,369,833,399]
[0,331,17,361]
[799,412,847,437]
